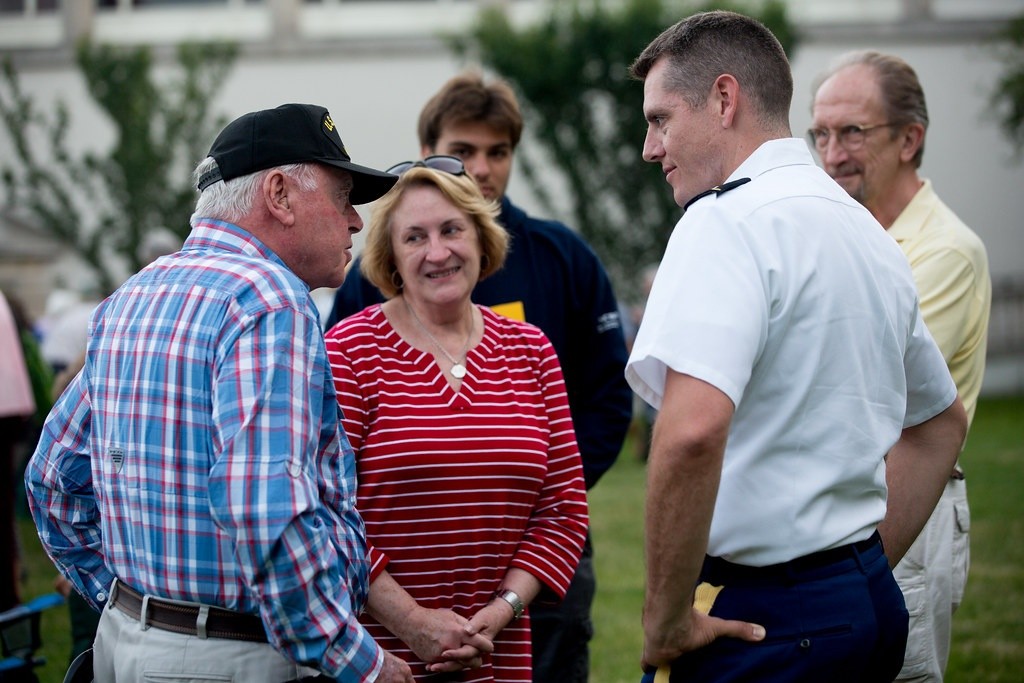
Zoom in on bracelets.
[490,589,524,620]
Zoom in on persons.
[806,55,994,683]
[24,104,413,682]
[624,10,967,683]
[321,76,634,683]
[324,158,589,682]
[1,285,100,680]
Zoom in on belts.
[110,581,271,644]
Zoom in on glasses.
[384,154,474,184]
[807,118,911,153]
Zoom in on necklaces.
[403,289,474,379]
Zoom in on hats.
[198,103,399,205]
